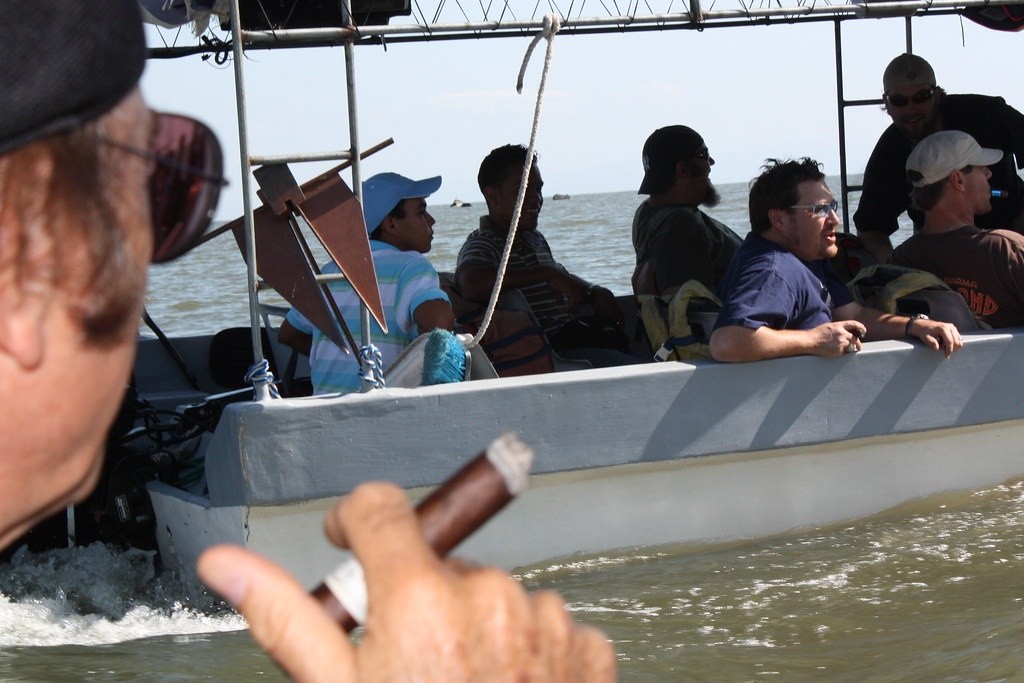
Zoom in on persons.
[887,131,1024,332]
[631,123,750,308]
[460,143,641,372]
[274,171,455,397]
[1,0,620,683]
[852,52,1024,266]
[707,158,963,360]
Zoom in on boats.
[14,0,1024,616]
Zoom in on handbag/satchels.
[554,314,631,352]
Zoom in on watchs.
[905,314,929,340]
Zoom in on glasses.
[888,84,937,107]
[55,113,229,262]
[680,147,709,164]
[783,201,837,218]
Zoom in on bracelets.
[587,283,600,298]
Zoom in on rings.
[848,342,854,354]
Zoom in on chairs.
[75,261,984,543]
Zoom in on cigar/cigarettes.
[311,432,532,631]
[850,327,865,338]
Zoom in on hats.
[363,173,442,236]
[882,53,935,95]
[0,0,146,155]
[905,130,1005,187]
[638,126,705,196]
